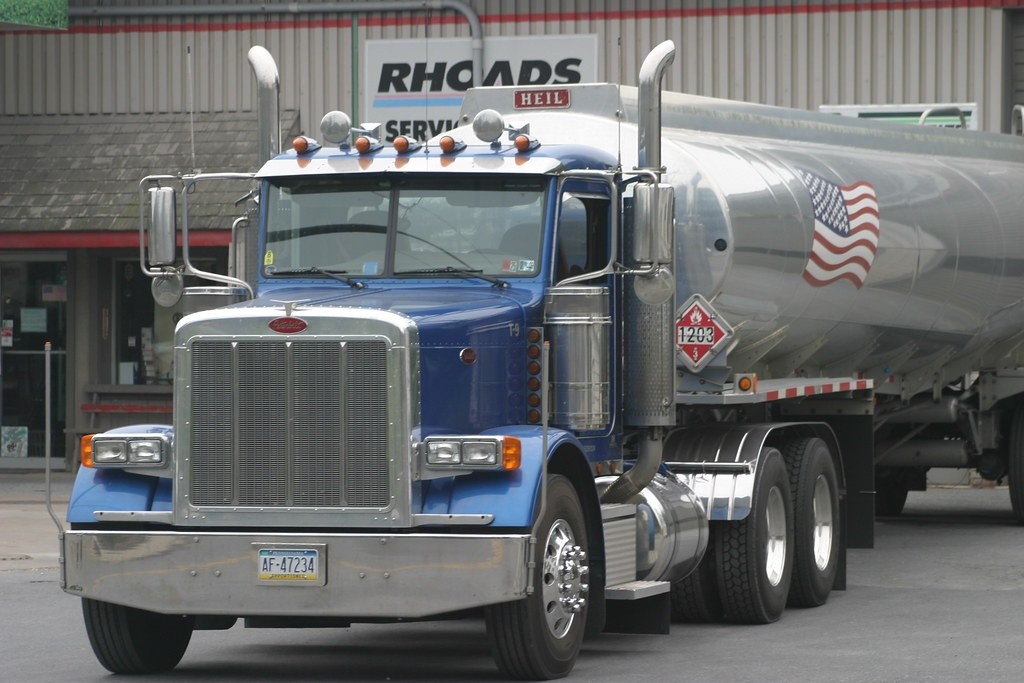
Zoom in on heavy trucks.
[45,35,1019,683]
